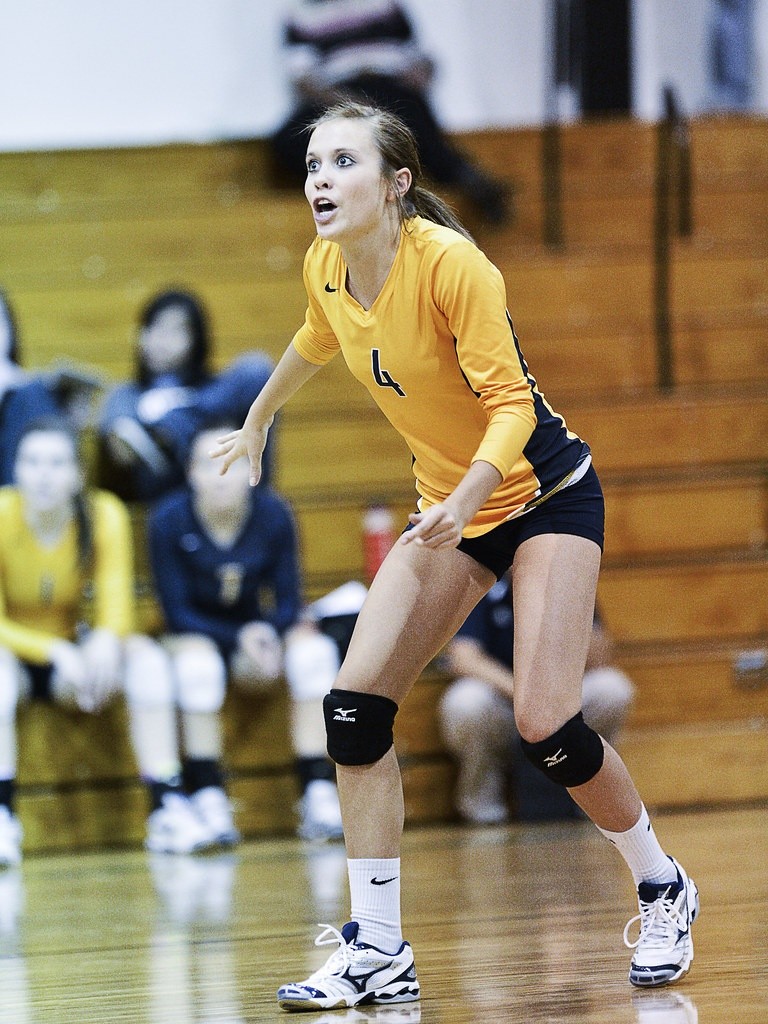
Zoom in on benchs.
[0,112,768,856]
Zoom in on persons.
[0,286,634,873]
[207,101,701,1008]
[261,1,514,227]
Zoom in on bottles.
[362,504,395,573]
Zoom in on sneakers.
[278,922,420,1010]
[624,855,699,988]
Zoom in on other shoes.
[299,779,343,838]
[0,805,23,866]
[191,788,241,850]
[144,794,214,852]
